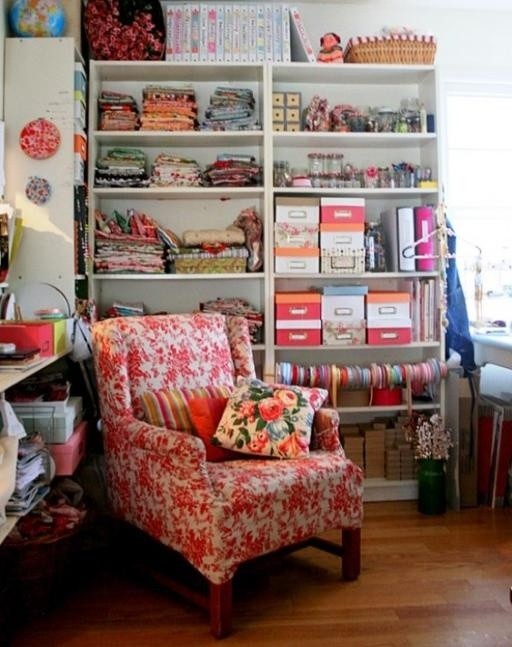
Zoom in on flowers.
[402,410,455,462]
[84,0,164,60]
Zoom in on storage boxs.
[49,421,87,478]
[0,323,54,360]
[272,195,416,346]
[11,395,85,442]
[5,318,65,356]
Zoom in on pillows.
[139,382,236,433]
[187,394,247,460]
[212,372,331,457]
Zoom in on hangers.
[401,205,484,261]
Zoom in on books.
[402,278,439,342]
[164,4,316,64]
[0,348,47,372]
[6,453,50,517]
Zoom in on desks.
[465,323,511,508]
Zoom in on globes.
[10,0,65,37]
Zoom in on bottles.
[399,95,420,131]
[308,152,431,188]
[420,102,427,134]
[365,221,386,271]
[378,107,393,132]
[274,160,291,187]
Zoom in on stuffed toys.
[318,32,344,63]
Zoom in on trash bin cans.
[2,537,72,621]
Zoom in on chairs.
[89,310,366,637]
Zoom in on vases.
[413,455,450,513]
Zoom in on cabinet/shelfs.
[89,58,270,384]
[2,36,89,325]
[0,341,84,545]
[270,61,449,500]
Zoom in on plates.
[20,118,62,160]
[25,178,51,205]
